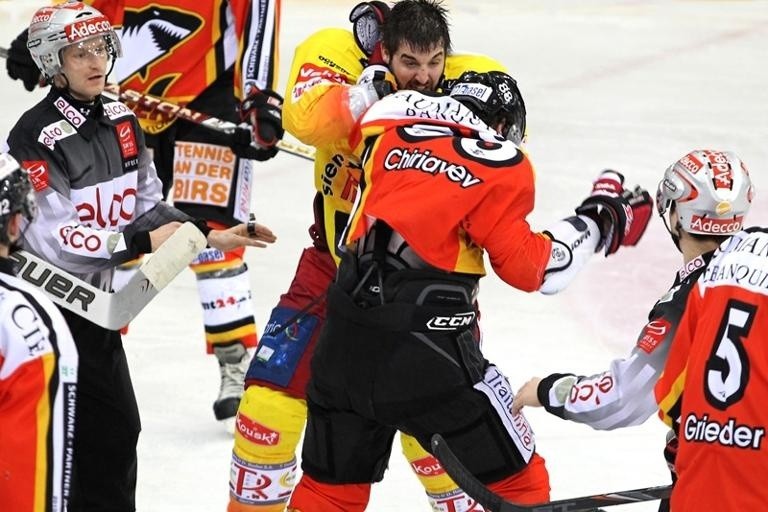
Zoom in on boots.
[208,339,254,421]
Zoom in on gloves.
[226,87,284,162]
[572,169,654,258]
[6,26,49,92]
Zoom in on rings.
[247,220,256,234]
[249,232,259,238]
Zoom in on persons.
[654,226,768,511]
[509,146,758,512]
[284,62,653,511]
[53,1,266,420]
[1,2,280,512]
[227,0,519,512]
[0,150,81,511]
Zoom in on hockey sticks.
[5,220,208,332]
[430,433,672,511]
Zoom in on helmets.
[0,150,40,244]
[654,144,756,239]
[447,67,526,149]
[26,1,124,81]
